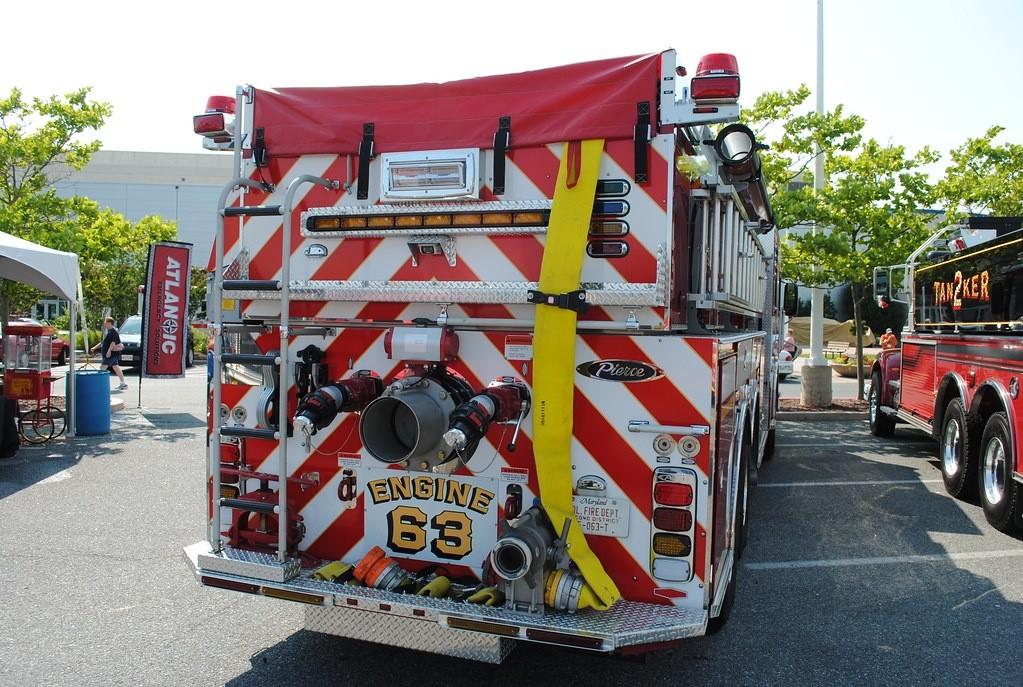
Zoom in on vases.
[831,363,871,378]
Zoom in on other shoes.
[115,385,128,390]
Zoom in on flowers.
[836,354,875,371]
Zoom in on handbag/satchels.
[111,343,125,351]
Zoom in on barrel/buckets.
[66,370,110,436]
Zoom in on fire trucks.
[866,216,1023,539]
[177,49,799,659]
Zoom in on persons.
[879,328,898,351]
[90,317,130,390]
[783,328,795,359]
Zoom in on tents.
[0,230,78,438]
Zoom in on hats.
[886,328,892,333]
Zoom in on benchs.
[823,341,850,359]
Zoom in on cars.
[0,316,70,368]
[106,314,195,372]
